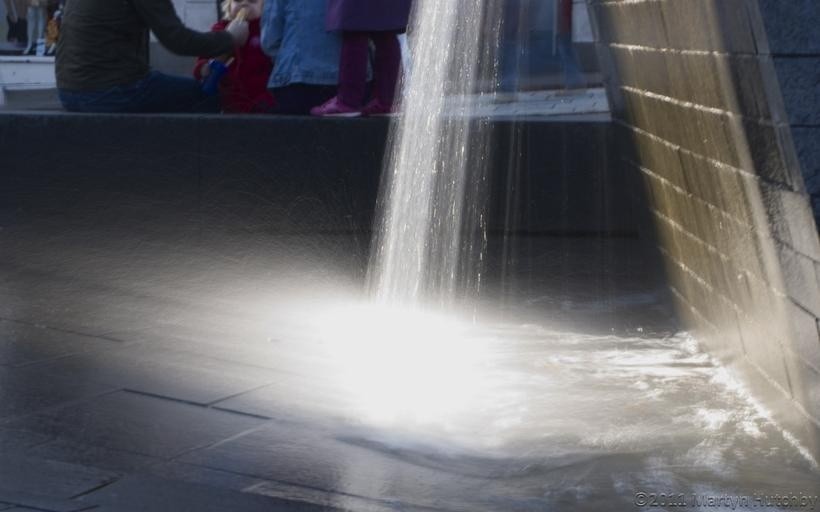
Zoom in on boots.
[6,14,28,47]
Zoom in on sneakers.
[362,98,406,118]
[23,43,57,56]
[310,96,362,118]
